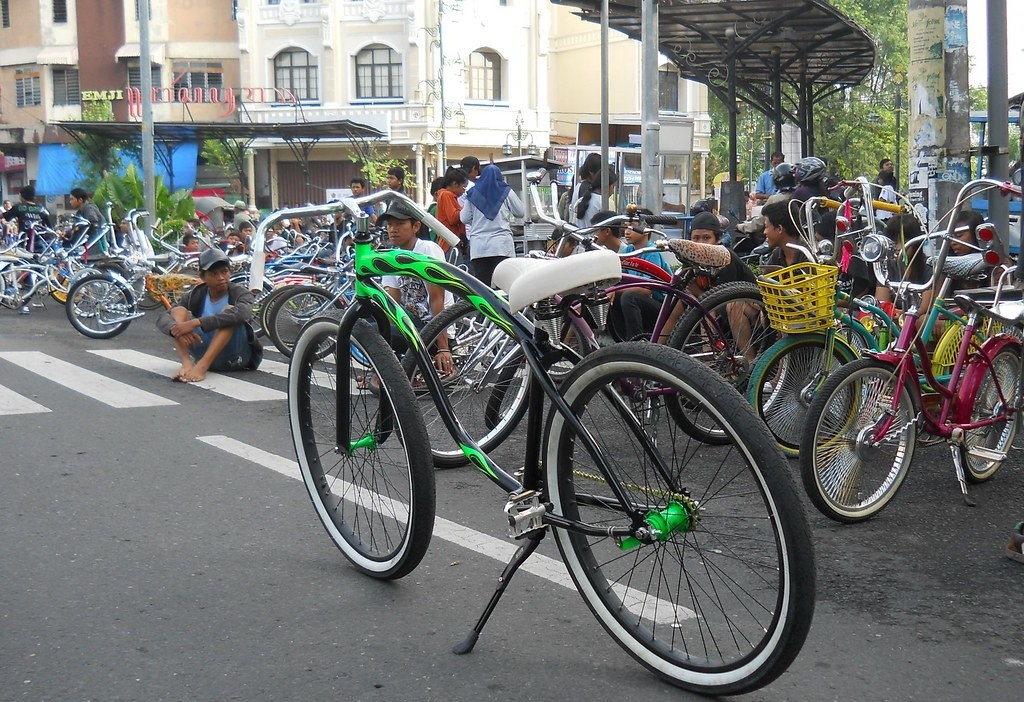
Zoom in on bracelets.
[437,349,450,352]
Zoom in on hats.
[691,212,721,230]
[376,200,419,226]
[234,200,246,210]
[199,248,231,270]
[459,156,481,176]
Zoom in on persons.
[459,165,525,290]
[156,248,263,383]
[425,156,481,274]
[553,152,1013,394]
[343,178,377,234]
[355,202,453,388]
[180,200,353,262]
[383,167,407,211]
[0,186,138,315]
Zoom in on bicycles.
[0,176,1024,526]
[248,190,818,697]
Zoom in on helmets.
[792,157,827,186]
[771,163,795,194]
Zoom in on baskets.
[756,262,837,334]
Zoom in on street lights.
[502,110,538,157]
